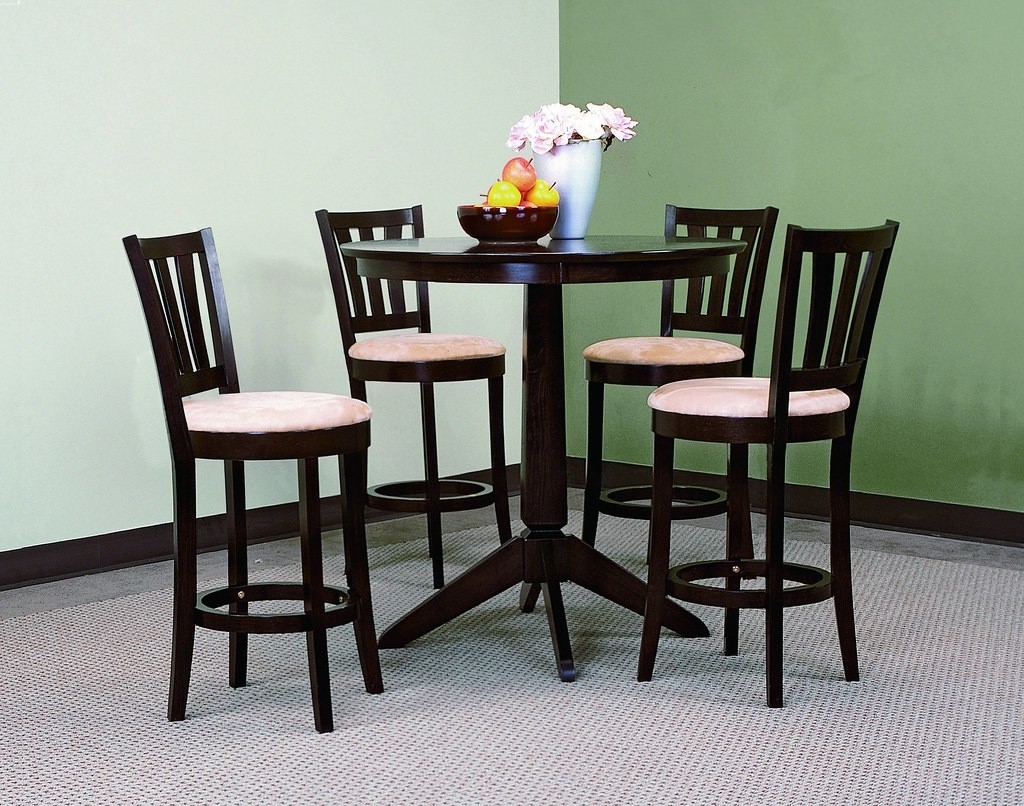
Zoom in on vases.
[533,143,603,240]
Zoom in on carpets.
[1,511,1024,806]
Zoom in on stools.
[637,219,903,702]
[316,207,513,589]
[126,226,385,733]
[580,205,779,560]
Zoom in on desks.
[340,237,745,684]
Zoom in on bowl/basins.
[457,205,560,245]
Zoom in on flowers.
[505,101,639,155]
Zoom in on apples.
[479,156,560,207]
